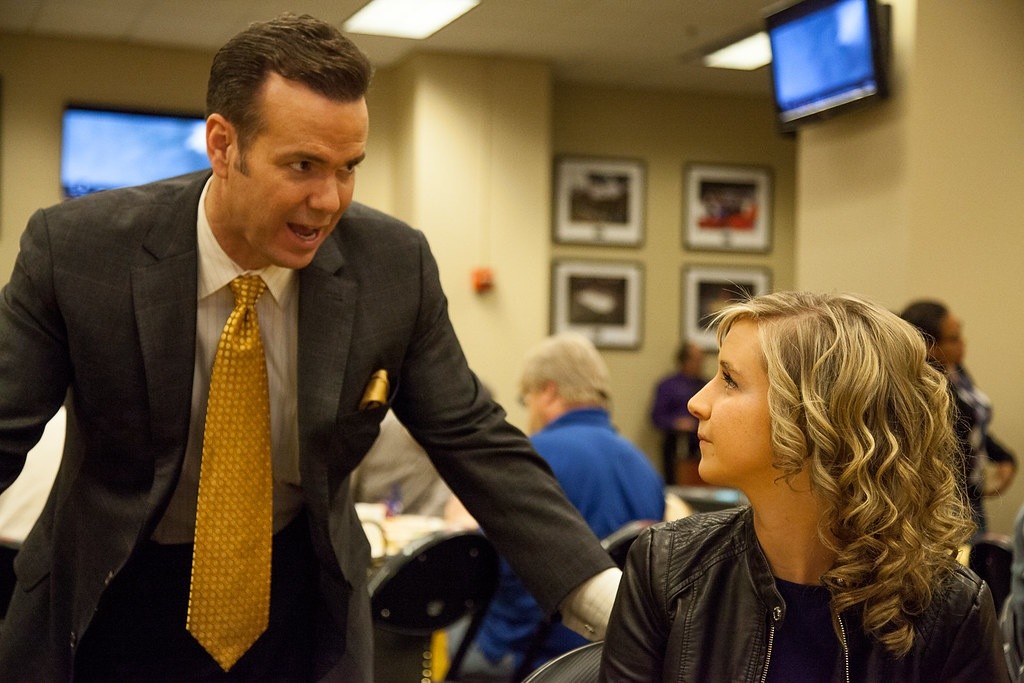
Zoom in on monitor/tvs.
[765,0,887,136]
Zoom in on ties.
[186,271,274,670]
